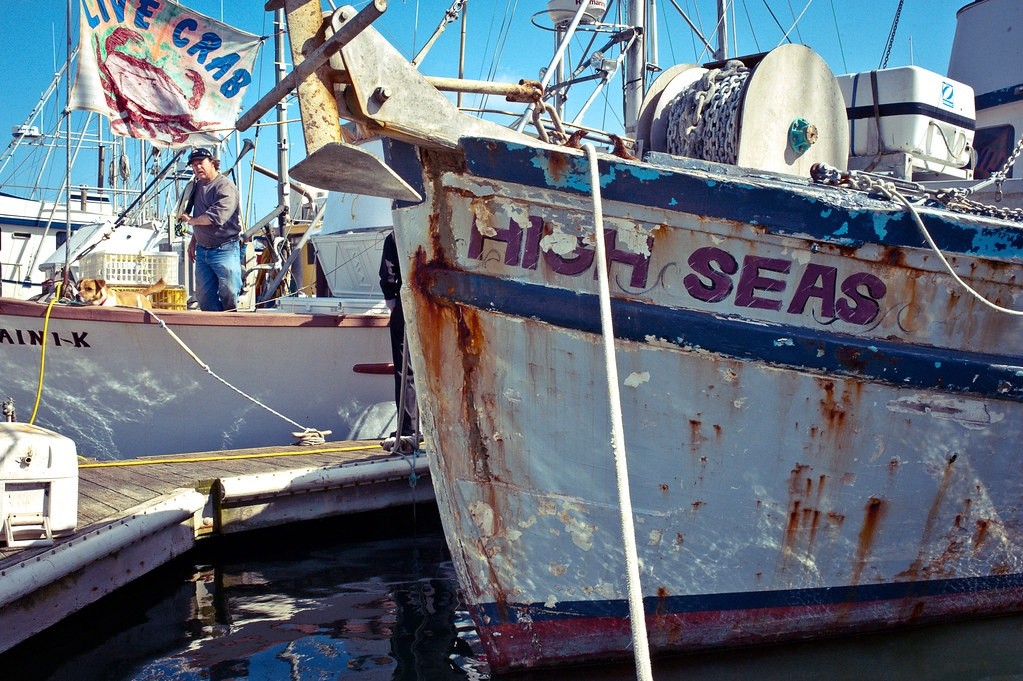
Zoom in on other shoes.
[390,430,424,443]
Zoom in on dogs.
[75,277,167,309]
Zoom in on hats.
[187,148,212,166]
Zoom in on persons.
[179,149,243,311]
[378,230,423,442]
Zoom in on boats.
[0,0,1023,681]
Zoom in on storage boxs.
[275,233,396,315]
[78,251,187,312]
